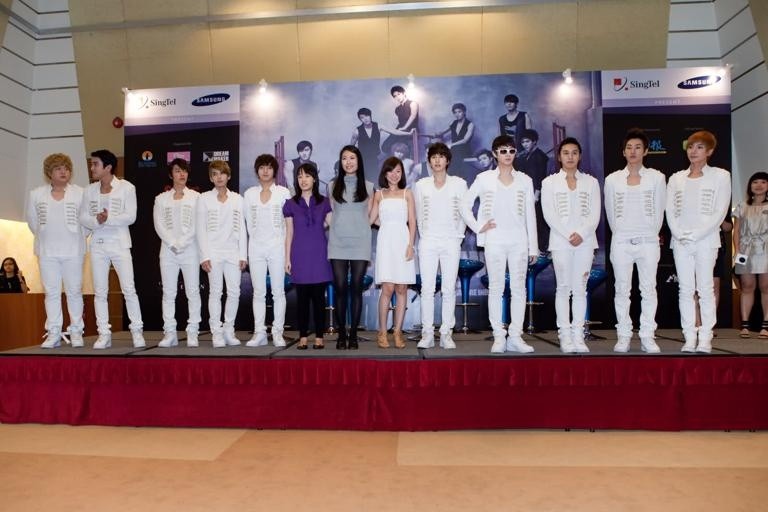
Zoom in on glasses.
[495,148,517,155]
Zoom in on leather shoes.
[299,329,359,350]
[378,330,405,350]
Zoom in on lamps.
[258,77,268,88]
[406,71,415,82]
[560,67,572,79]
[119,83,129,95]
[719,60,733,76]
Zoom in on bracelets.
[408,244,415,250]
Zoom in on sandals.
[758,321,768,339]
[740,320,750,338]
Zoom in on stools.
[250,252,607,346]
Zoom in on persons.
[280,85,554,274]
[25,152,90,348]
[457,134,541,354]
[0,257,30,294]
[541,136,601,353]
[323,145,379,352]
[196,159,249,349]
[410,141,468,350]
[666,131,732,354]
[694,203,733,338]
[282,162,333,350]
[152,157,202,349]
[602,128,666,355]
[79,150,146,348]
[368,156,416,348]
[730,172,768,340]
[244,154,292,349]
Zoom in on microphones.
[734,253,748,265]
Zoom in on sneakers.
[131,330,146,347]
[680,335,712,352]
[159,332,286,348]
[40,327,112,350]
[641,337,660,353]
[560,335,589,354]
[492,337,534,353]
[416,334,456,348]
[614,337,631,353]
